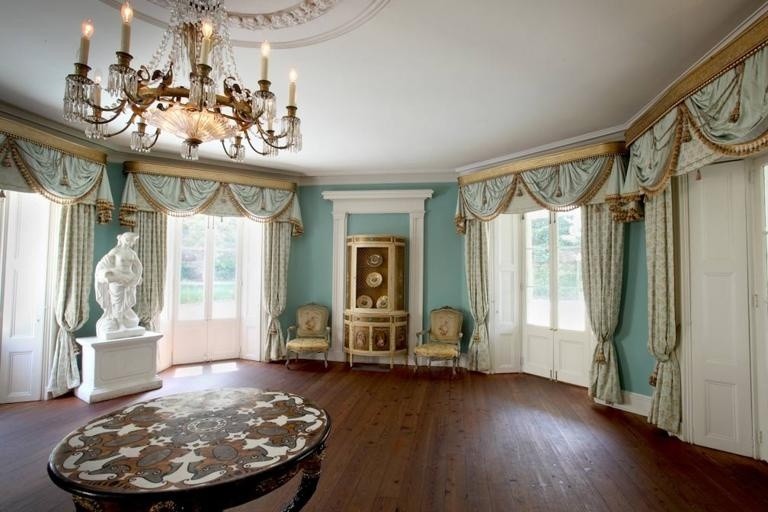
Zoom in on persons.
[96,231,141,332]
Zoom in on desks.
[47,388,331,511]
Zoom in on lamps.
[62,0,303,162]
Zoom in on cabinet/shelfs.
[343,234,410,369]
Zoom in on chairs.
[413,305,465,375]
[285,303,331,369]
